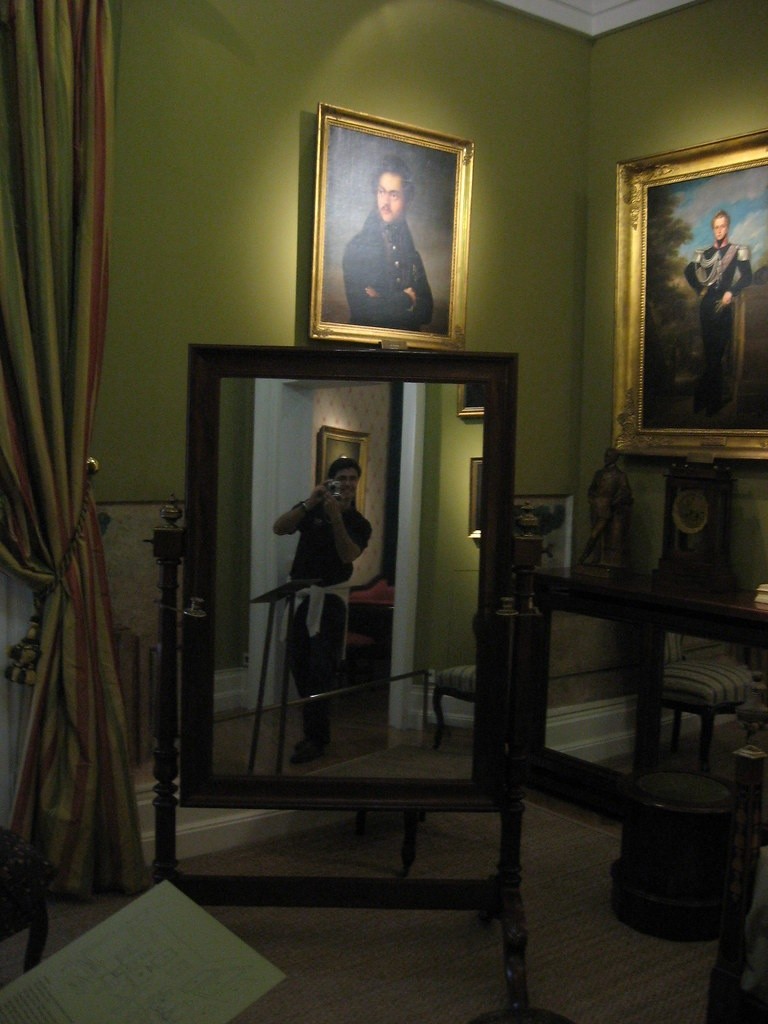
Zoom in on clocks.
[652,458,737,594]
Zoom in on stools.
[428,667,476,749]
[612,766,736,940]
[660,660,757,781]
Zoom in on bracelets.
[299,501,310,512]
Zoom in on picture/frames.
[317,425,369,517]
[456,383,484,417]
[612,130,768,463]
[468,457,482,540]
[306,102,474,352]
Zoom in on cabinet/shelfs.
[518,571,768,830]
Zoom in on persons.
[573,448,632,568]
[272,457,372,765]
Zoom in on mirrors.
[175,345,519,812]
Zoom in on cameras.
[326,481,341,497]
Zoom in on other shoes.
[294,734,324,761]
